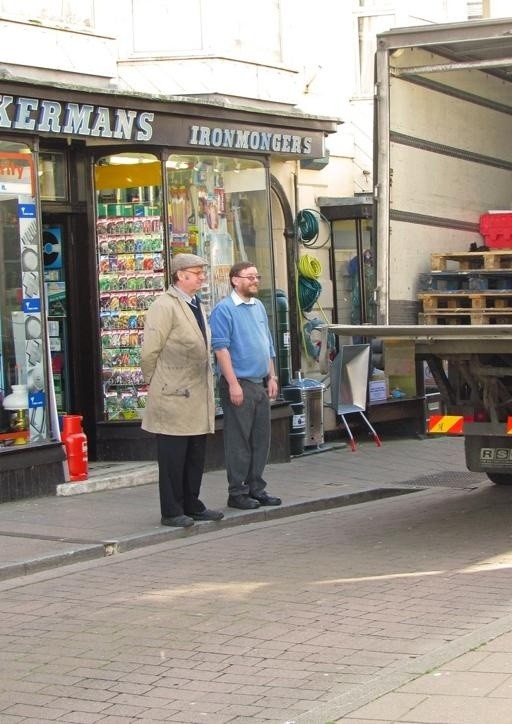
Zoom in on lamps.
[1,384,30,445]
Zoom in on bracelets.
[268,374,279,381]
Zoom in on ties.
[191,299,196,306]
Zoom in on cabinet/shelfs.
[90,206,220,463]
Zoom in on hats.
[172,254,208,274]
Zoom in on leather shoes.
[252,492,281,505]
[187,510,223,520]
[227,494,259,508]
[161,516,193,526]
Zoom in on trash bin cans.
[280,371,324,450]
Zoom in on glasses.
[183,269,205,277]
[238,276,261,281]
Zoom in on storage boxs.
[479,211,512,249]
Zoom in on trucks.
[314,14,512,484]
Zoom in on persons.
[140,252,228,526]
[212,262,284,511]
